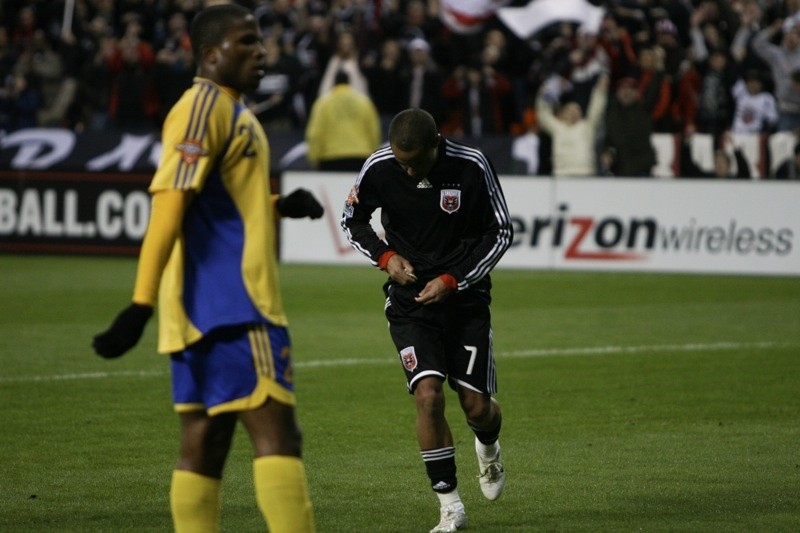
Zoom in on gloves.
[93,304,154,359]
[277,189,324,220]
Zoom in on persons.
[0,0,799,179]
[88,5,325,532]
[332,107,509,533]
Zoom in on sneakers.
[429,506,466,533]
[475,439,505,501]
